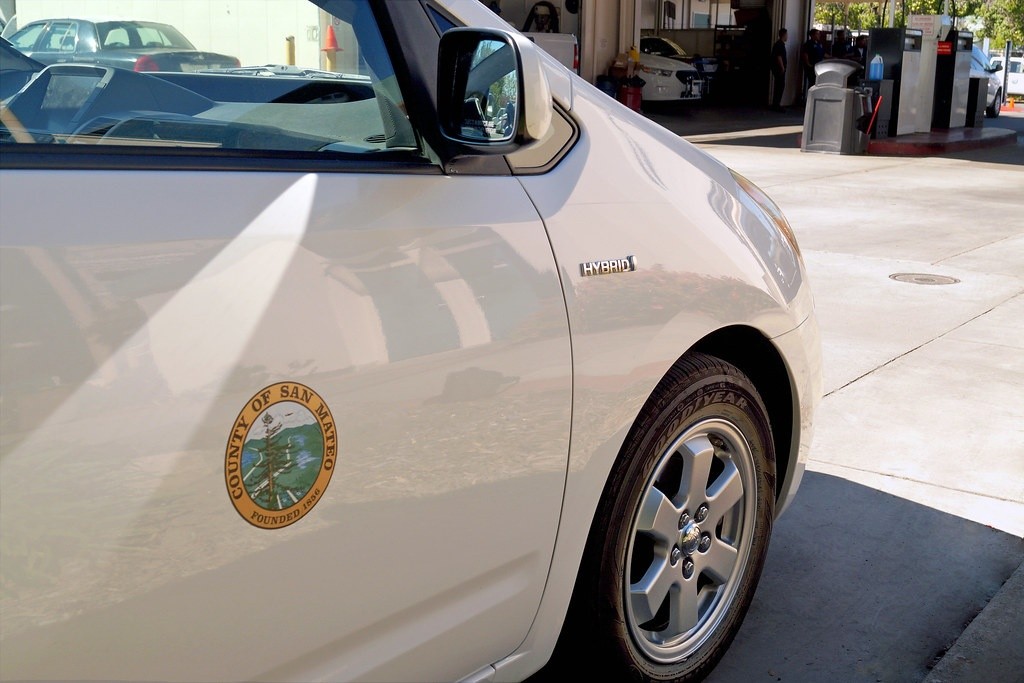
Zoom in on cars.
[0,0,823,682]
[991,57,1024,106]
[639,34,705,108]
[9,18,244,74]
[970,44,1004,117]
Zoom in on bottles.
[869,54,883,81]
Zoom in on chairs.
[59,36,75,51]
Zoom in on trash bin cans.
[865,29,990,139]
[800,59,872,155]
[595,75,642,114]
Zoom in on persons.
[801,29,832,89]
[770,29,788,113]
[829,31,867,66]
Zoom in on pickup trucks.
[521,30,580,76]
